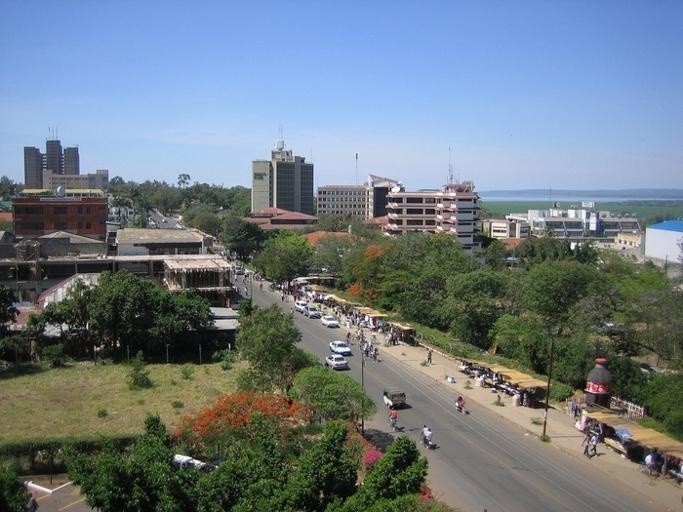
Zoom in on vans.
[235,267,241,274]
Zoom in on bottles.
[585,358,612,409]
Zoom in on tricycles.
[382,391,406,409]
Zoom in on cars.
[329,340,352,355]
[325,353,349,369]
[294,300,338,328]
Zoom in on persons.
[387,407,398,426]
[582,432,599,459]
[242,275,399,361]
[422,425,432,445]
[427,349,432,364]
[643,450,656,477]
[456,395,463,406]
